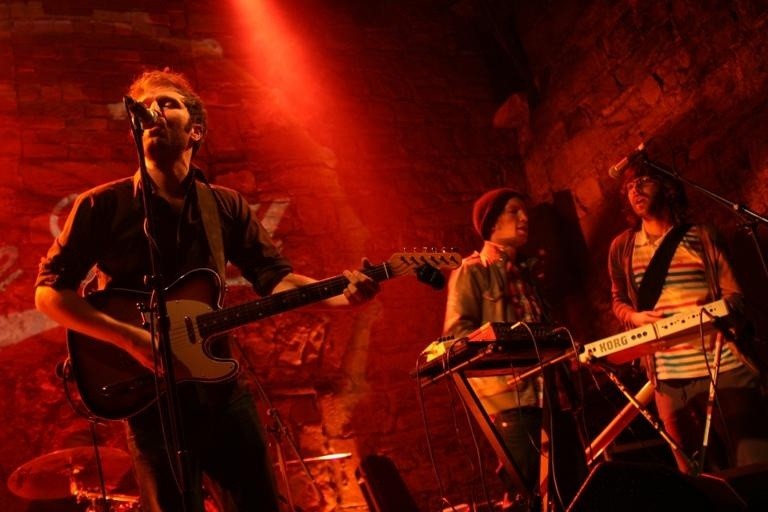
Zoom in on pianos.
[410,320,585,385]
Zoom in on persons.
[33,66,380,512]
[443,187,589,511]
[607,149,768,477]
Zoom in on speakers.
[566,460,752,512]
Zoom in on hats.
[471,186,526,240]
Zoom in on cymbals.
[8,447,133,500]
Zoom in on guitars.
[64,248,466,420]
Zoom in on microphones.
[126,97,159,129]
[608,142,647,180]
